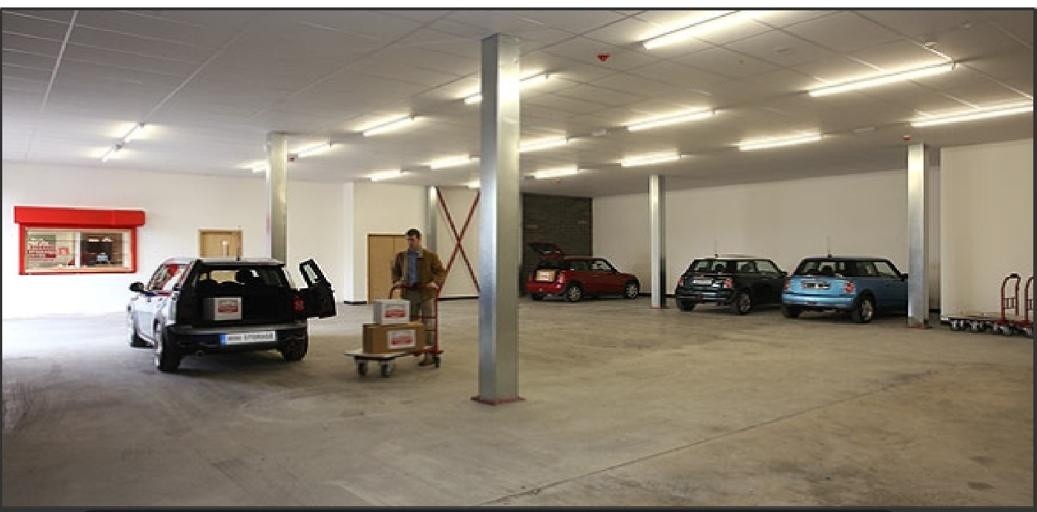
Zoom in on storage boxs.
[364,322,425,354]
[203,298,243,322]
[373,298,408,325]
[536,268,559,282]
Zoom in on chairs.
[233,271,252,284]
[821,265,834,274]
[715,263,727,271]
[742,264,755,273]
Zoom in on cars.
[780,255,908,324]
[675,258,790,316]
[527,255,639,303]
[125,257,336,372]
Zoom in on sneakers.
[417,352,434,365]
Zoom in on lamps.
[905,97,1033,134]
[424,67,586,189]
[806,56,966,102]
[739,127,825,158]
[638,12,754,51]
[101,116,149,169]
[617,148,687,169]
[245,104,421,184]
[625,105,721,135]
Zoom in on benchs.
[194,278,264,316]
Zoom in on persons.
[390,229,445,366]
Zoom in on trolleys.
[343,284,439,378]
[948,273,1021,332]
[977,275,1036,337]
